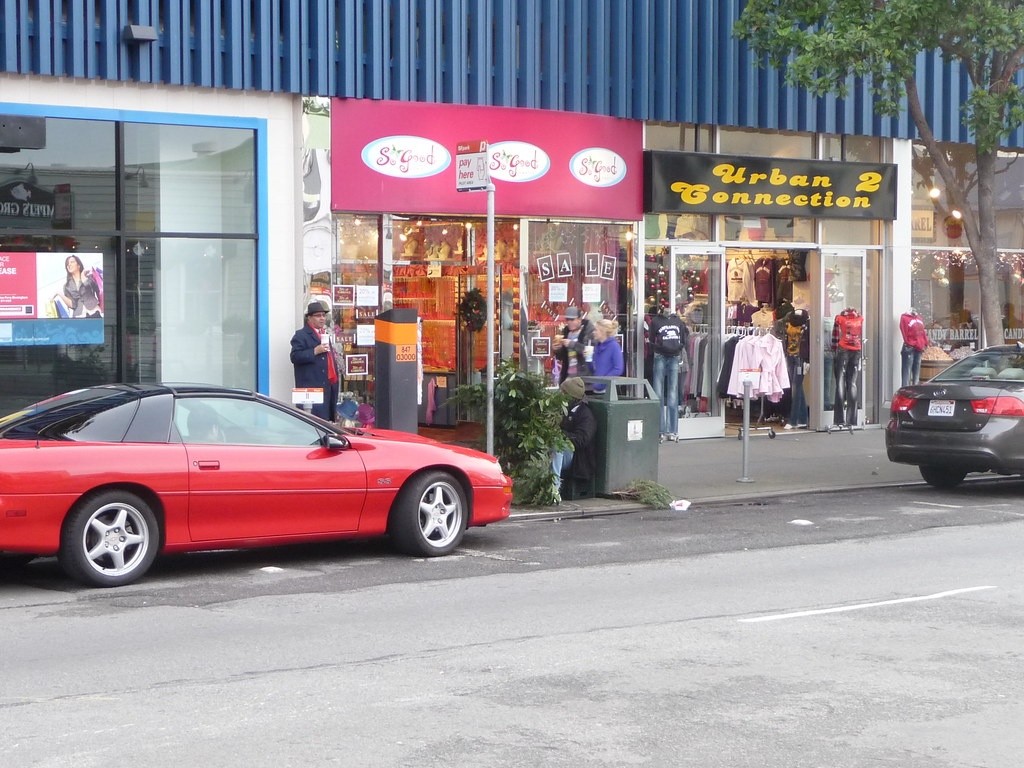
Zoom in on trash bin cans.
[580,376,661,495]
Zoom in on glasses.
[567,318,575,321]
[310,313,326,318]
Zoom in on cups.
[554,335,564,346]
[321,333,330,348]
[585,346,595,363]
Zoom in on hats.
[356,403,375,423]
[305,302,328,316]
[563,306,581,319]
[560,376,585,399]
[334,398,360,420]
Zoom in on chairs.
[971,367,997,377]
[997,368,1024,379]
[185,406,220,444]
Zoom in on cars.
[1,383,513,587]
[886,341,1024,488]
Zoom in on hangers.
[690,323,782,342]
[741,297,752,306]
[760,302,771,315]
[760,250,778,260]
[694,292,730,306]
[736,249,752,262]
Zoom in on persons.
[899,307,928,388]
[51,255,104,319]
[550,306,598,387]
[650,308,689,442]
[289,303,338,422]
[782,309,811,429]
[832,305,863,425]
[582,319,623,394]
[949,309,979,352]
[1001,304,1024,344]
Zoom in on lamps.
[16,162,39,186]
[400,228,413,242]
[126,167,149,189]
[124,24,159,42]
[442,226,451,234]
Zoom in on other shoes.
[551,490,562,503]
